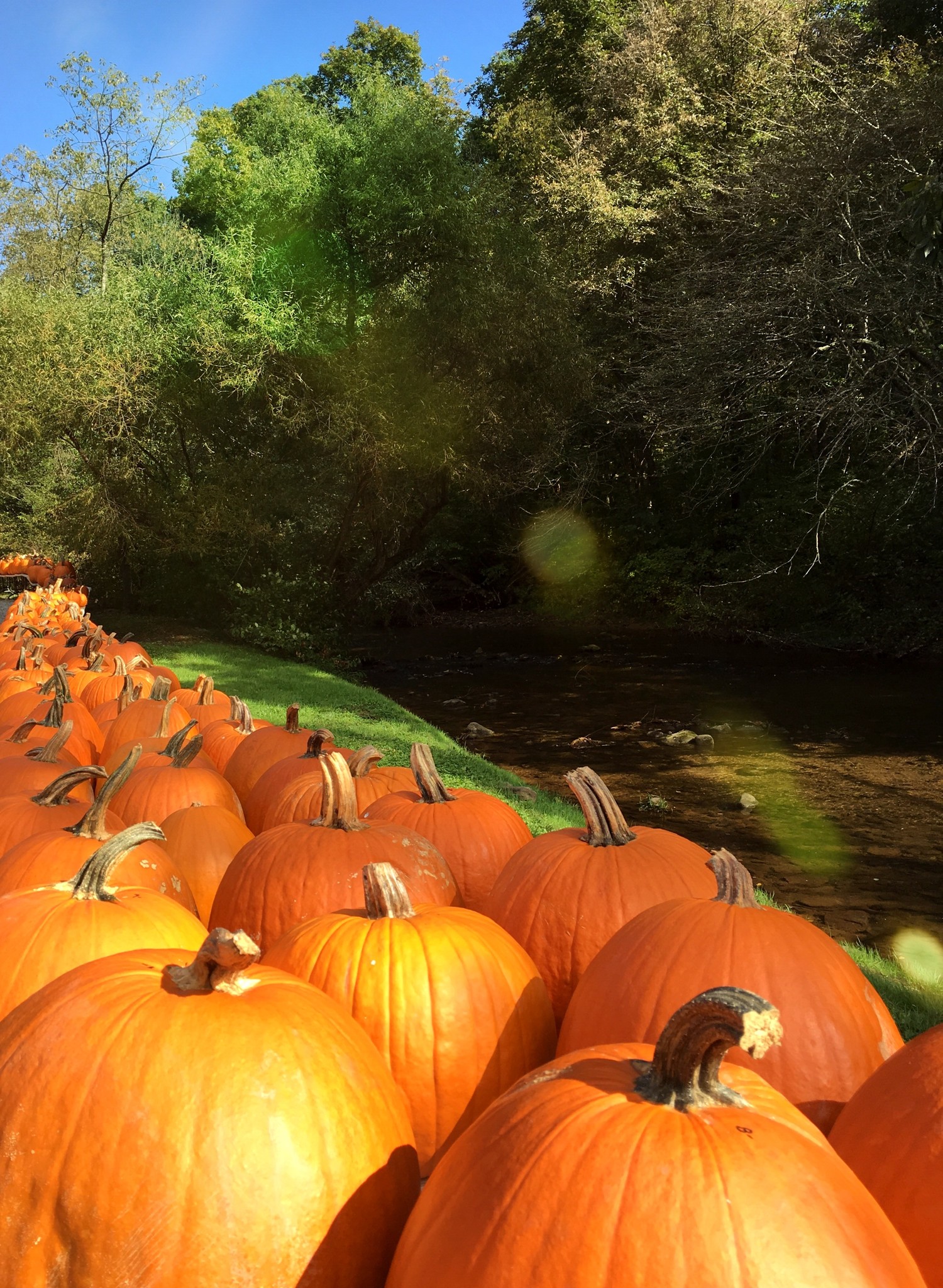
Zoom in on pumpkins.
[0,550,943,1288]
